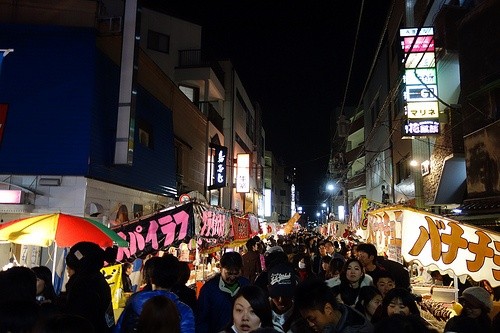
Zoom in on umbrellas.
[0,212,131,249]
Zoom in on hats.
[266,265,297,297]
[457,287,491,312]
[67,242,104,271]
[31,266,52,281]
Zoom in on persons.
[0,224,494,333]
